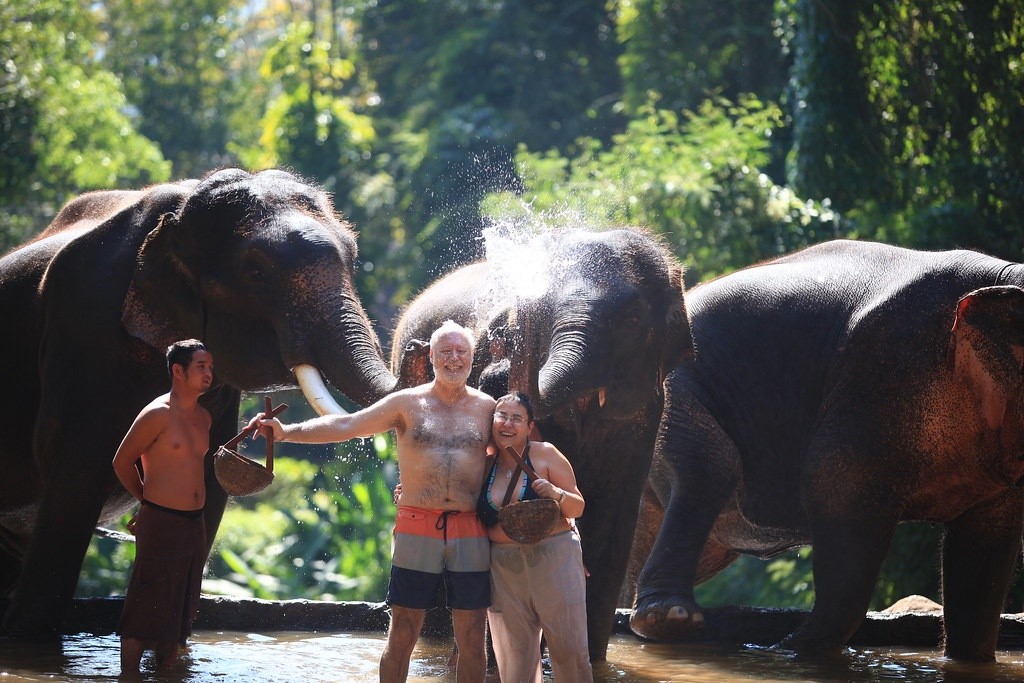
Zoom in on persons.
[113,339,213,676]
[242,322,581,683]
[394,394,594,683]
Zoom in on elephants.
[388,219,1023,666]
[1,164,436,674]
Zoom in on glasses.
[492,412,529,426]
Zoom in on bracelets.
[559,489,566,503]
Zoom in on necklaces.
[500,457,512,477]
